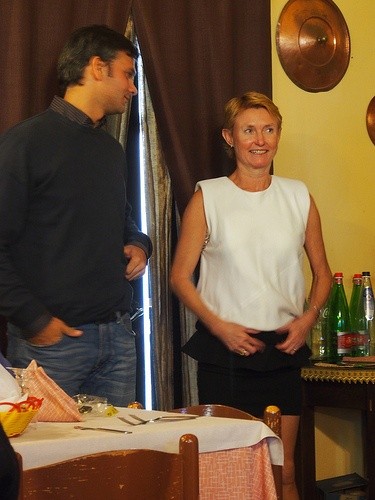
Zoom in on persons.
[0,26,151,410]
[171,91,334,500]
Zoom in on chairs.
[15,434,201,500]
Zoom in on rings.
[293,348,298,352]
[243,350,247,356]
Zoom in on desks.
[299,365,375,500]
[9,406,284,500]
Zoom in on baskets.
[0,397,44,436]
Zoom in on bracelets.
[308,307,320,318]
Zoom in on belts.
[96,310,125,323]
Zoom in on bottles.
[309,301,328,362]
[358,271,375,358]
[349,274,370,357]
[326,272,350,364]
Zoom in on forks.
[116,414,201,425]
